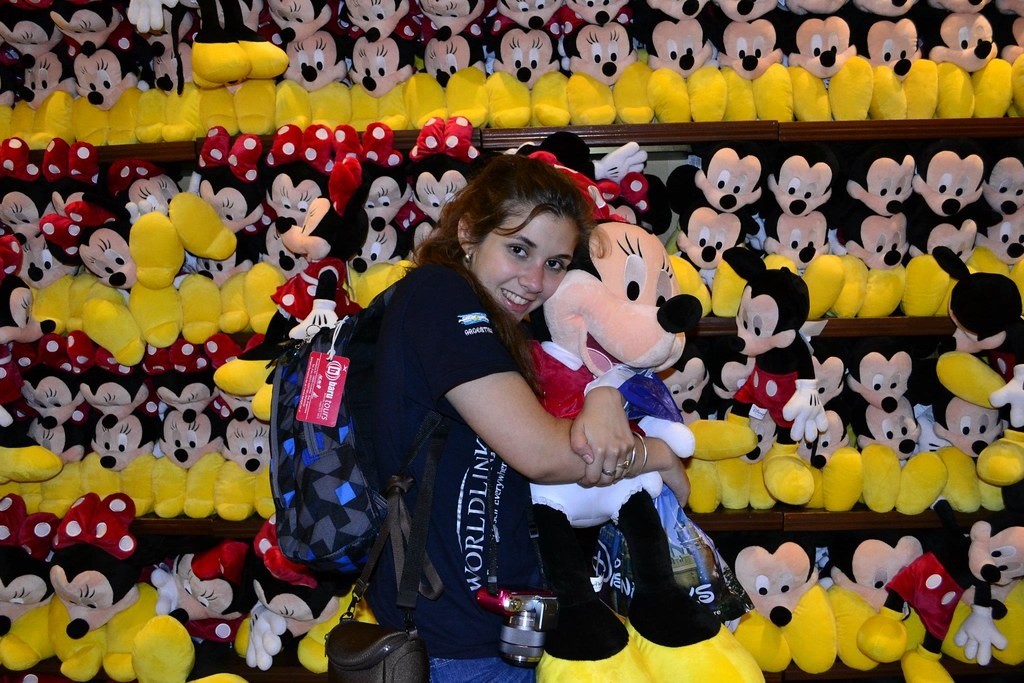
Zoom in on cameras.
[476,587,559,669]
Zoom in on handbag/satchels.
[324,620,429,683]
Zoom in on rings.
[617,461,629,468]
[602,469,616,475]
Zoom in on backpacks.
[268,276,446,583]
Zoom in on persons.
[344,154,691,683]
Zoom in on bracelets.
[631,431,647,478]
[628,447,636,469]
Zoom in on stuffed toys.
[513,144,765,682]
[1,117,1024,683]
[0,0,1024,149]
